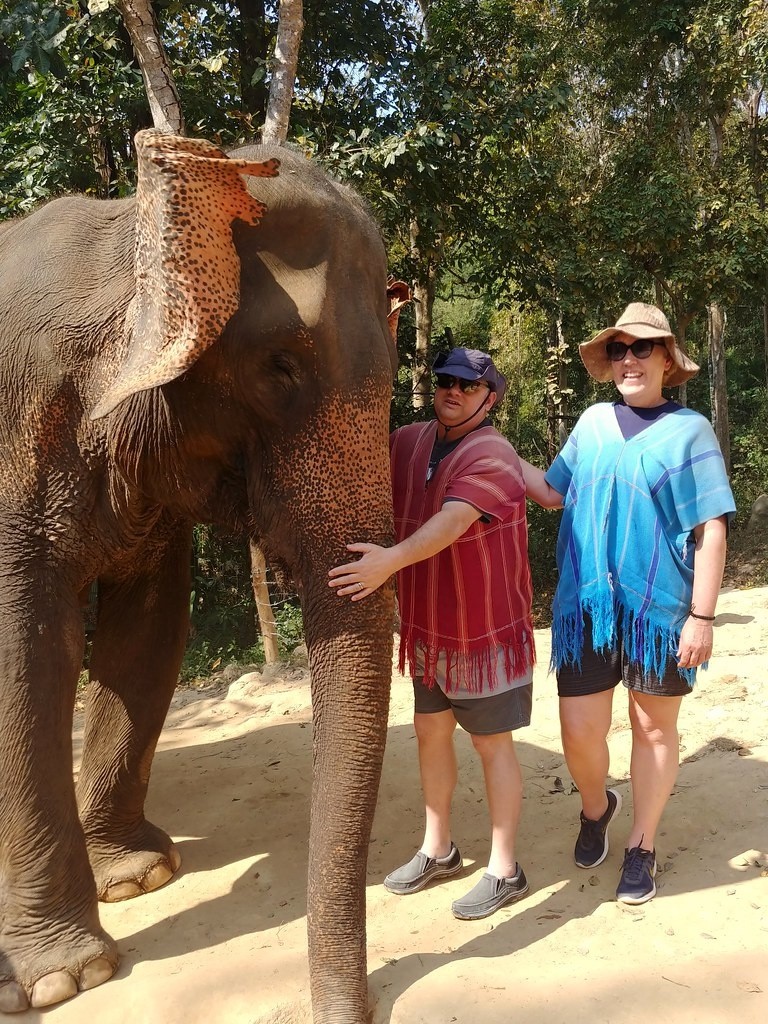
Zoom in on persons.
[326,347,537,920]
[517,302,737,906]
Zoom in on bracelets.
[687,610,717,621]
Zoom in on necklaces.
[426,438,460,481]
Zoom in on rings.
[357,582,364,589]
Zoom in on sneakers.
[573,790,621,868]
[451,860,528,920]
[615,845,659,904]
[383,840,462,895]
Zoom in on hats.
[432,347,506,408]
[579,302,701,388]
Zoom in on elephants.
[0,126,411,1024]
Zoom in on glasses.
[436,372,492,394]
[605,338,666,361]
